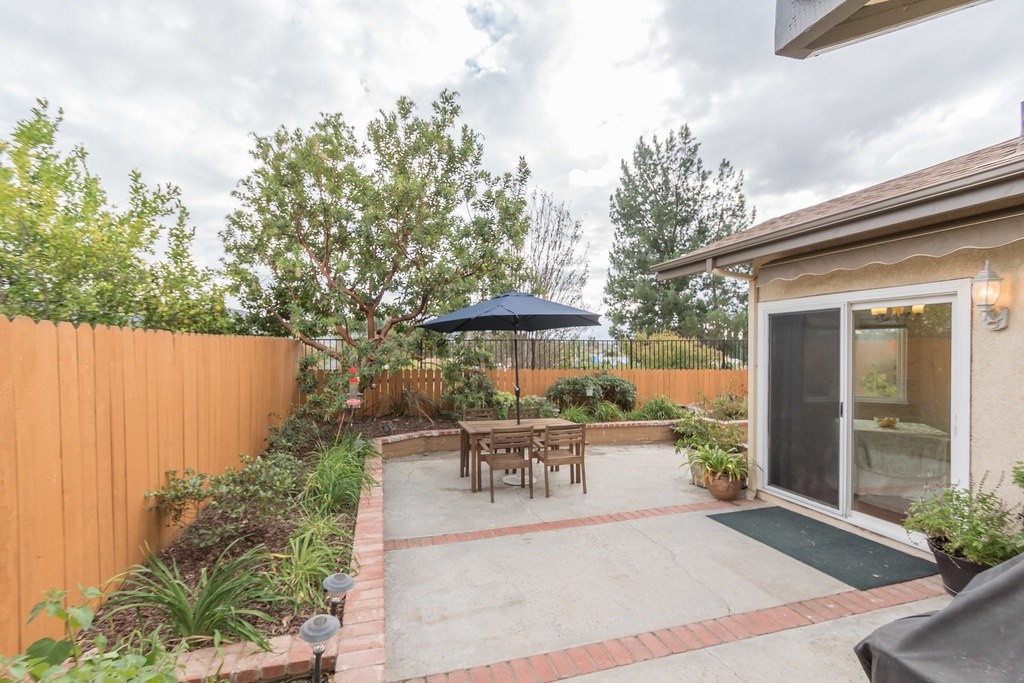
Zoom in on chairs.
[507,406,554,471]
[534,423,588,498]
[478,424,535,503]
[462,407,510,476]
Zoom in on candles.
[672,408,754,502]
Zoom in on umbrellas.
[413,291,602,425]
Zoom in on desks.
[834,414,947,450]
[457,417,581,493]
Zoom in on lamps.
[583,382,596,399]
[321,572,353,610]
[969,258,1008,332]
[300,612,339,657]
[871,302,930,323]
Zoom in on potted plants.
[901,466,1024,599]
